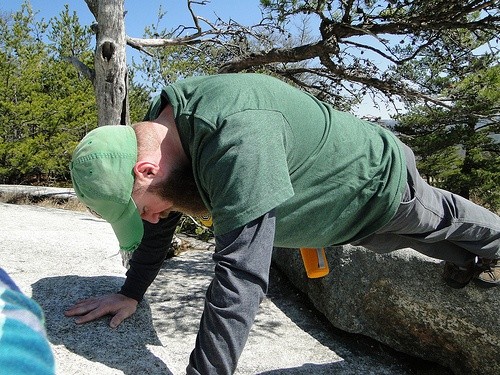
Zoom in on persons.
[63,70,500,375]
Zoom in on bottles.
[300,248,329,279]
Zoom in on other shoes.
[445,258,499,291]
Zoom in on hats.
[69,124,144,251]
